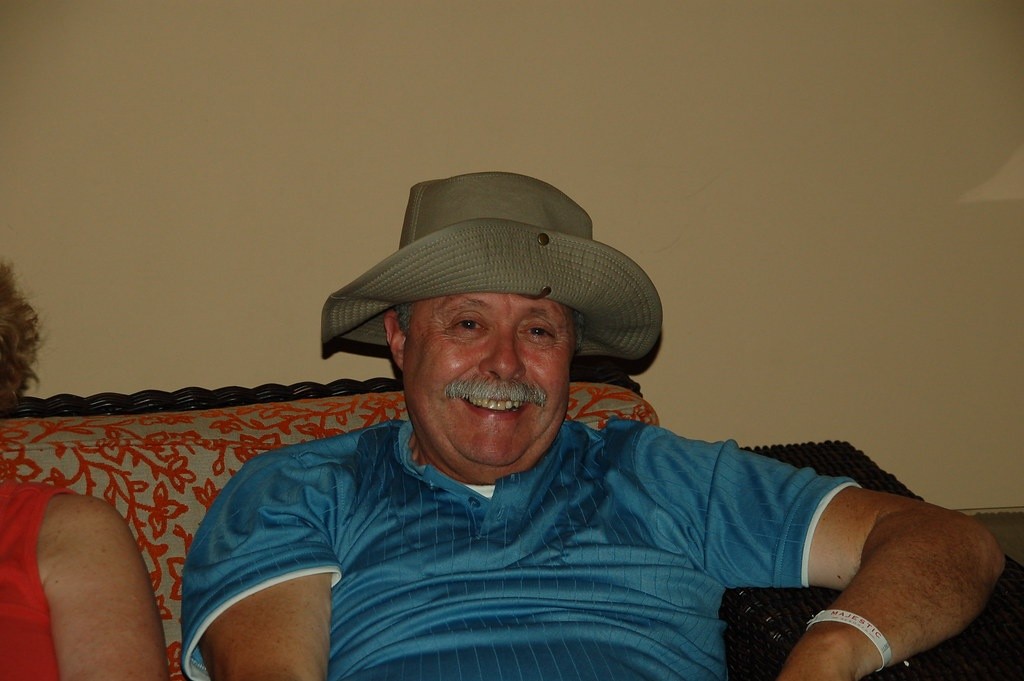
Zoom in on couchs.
[0,359,1023,681]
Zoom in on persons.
[0,260,171,681]
[180,171,1007,681]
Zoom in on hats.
[321,172,663,360]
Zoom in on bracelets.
[806,609,892,672]
